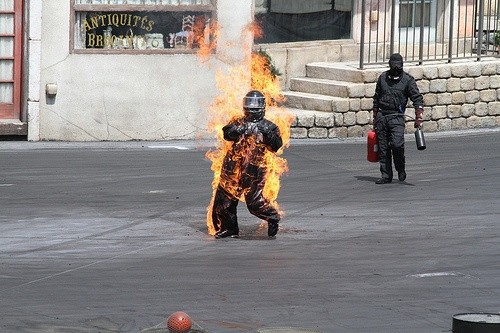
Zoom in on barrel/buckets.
[452,312,500,333]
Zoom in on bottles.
[415,128,426,150]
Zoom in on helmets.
[244,90,266,120]
[389,53,403,74]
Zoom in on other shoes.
[268,222,279,237]
[375,176,393,184]
[214,228,239,239]
[398,171,406,180]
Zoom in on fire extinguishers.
[367,113,427,163]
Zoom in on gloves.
[257,124,272,140]
[240,122,252,133]
[412,106,424,127]
[373,110,376,126]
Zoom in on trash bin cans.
[452,311,500,333]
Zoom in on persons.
[211,90,283,239]
[373,53,424,184]
